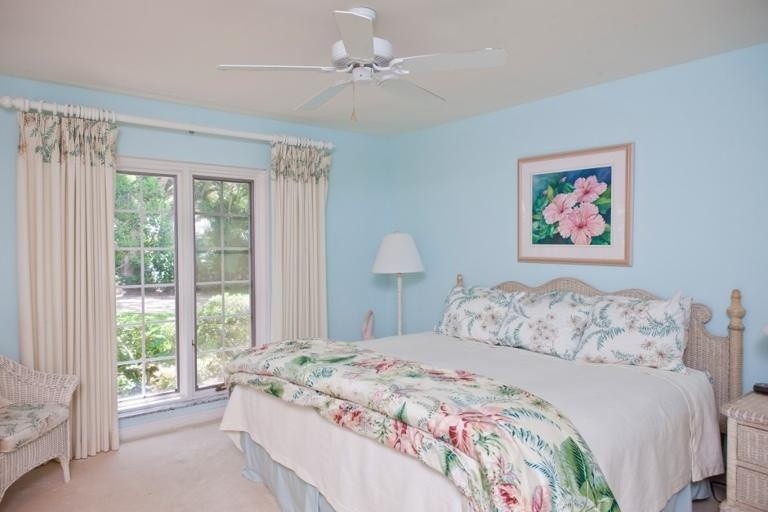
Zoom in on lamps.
[349,62,404,123]
[371,232,426,335]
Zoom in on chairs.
[0,353,82,505]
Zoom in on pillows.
[574,289,694,376]
[497,289,596,360]
[433,283,516,346]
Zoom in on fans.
[216,8,501,110]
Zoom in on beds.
[220,274,747,511]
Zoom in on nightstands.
[718,389,768,512]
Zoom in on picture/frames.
[517,141,636,266]
